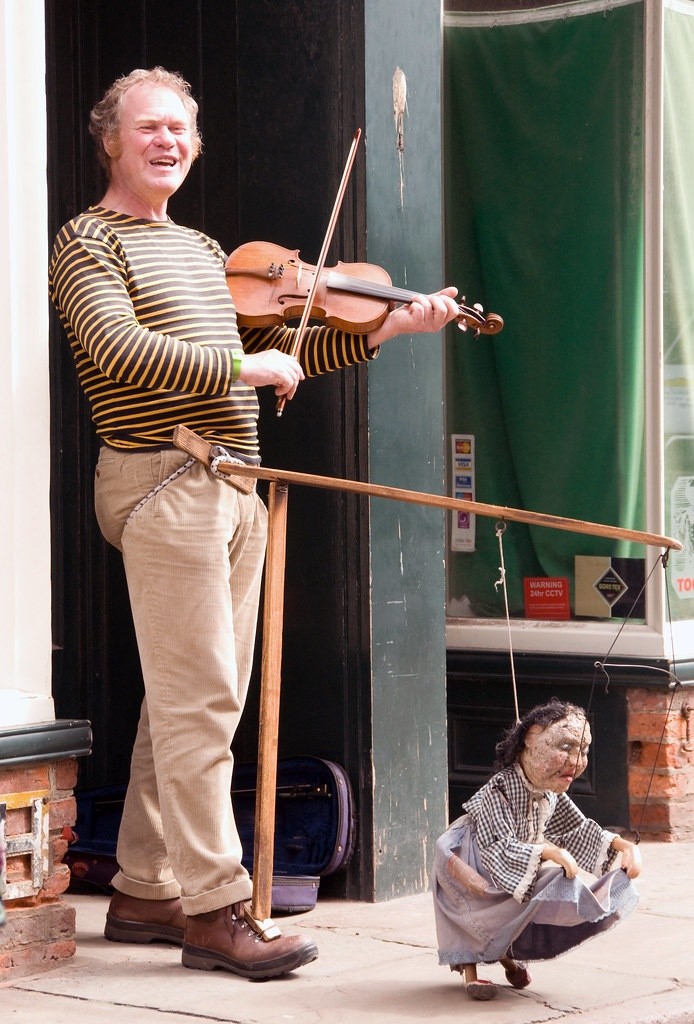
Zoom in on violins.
[226,237,508,338]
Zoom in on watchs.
[229,348,242,385]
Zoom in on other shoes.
[182,900,318,978]
[104,889,186,948]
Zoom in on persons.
[434,699,641,1000]
[49,66,457,978]
